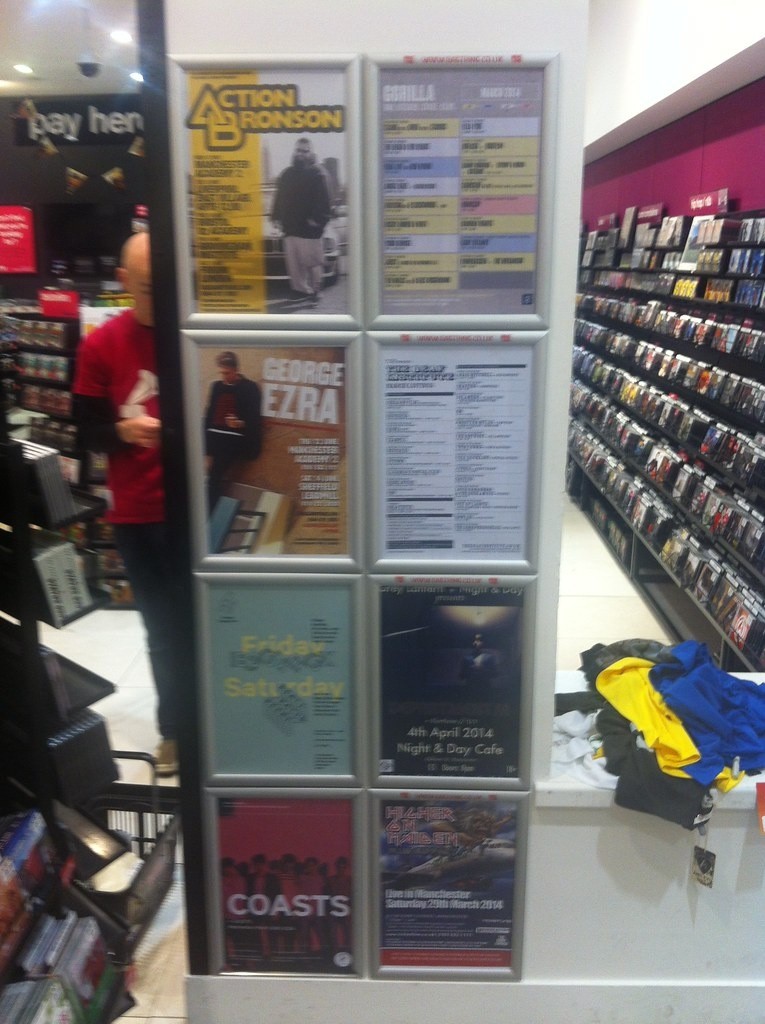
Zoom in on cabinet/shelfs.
[0,428,181,1024]
[567,210,765,673]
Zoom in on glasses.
[296,148,310,154]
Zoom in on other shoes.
[287,292,309,302]
[306,290,318,306]
[155,737,181,775]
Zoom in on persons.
[71,233,179,775]
[221,852,351,969]
[202,351,263,514]
[467,633,496,695]
[269,137,332,306]
